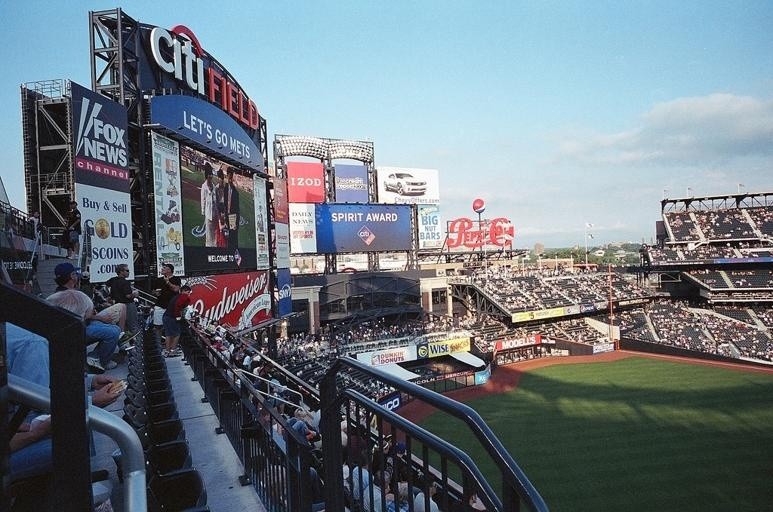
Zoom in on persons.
[222,167,241,249]
[414,472,442,512]
[26,211,40,239]
[412,258,773,380]
[634,201,772,257]
[2,289,126,485]
[64,200,82,260]
[4,208,18,236]
[185,307,414,512]
[199,161,216,247]
[9,412,54,484]
[454,488,483,512]
[214,167,229,247]
[42,261,193,374]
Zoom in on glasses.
[121,270,129,272]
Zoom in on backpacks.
[68,231,78,242]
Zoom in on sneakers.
[164,350,182,358]
[105,361,117,370]
[86,357,104,374]
[68,255,78,259]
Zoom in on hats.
[54,262,80,275]
[161,262,173,273]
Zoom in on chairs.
[116,319,394,512]
[462,210,773,362]
